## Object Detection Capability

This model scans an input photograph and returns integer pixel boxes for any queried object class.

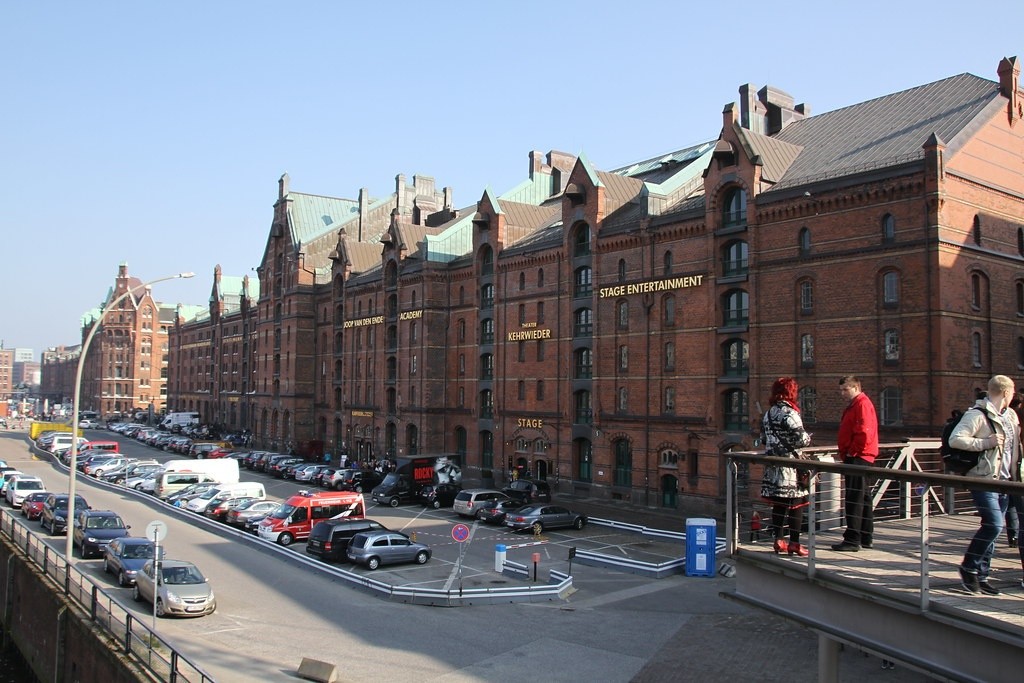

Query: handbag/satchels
[796,456,819,490]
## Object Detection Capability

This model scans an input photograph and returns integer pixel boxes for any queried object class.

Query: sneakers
[979,582,999,595]
[958,567,980,592]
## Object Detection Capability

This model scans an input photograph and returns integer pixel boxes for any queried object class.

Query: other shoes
[1008,537,1018,548]
[832,539,861,551]
[862,542,873,547]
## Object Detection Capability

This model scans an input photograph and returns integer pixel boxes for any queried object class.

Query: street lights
[65,272,196,562]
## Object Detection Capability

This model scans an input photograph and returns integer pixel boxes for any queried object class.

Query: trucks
[158,412,199,429]
[372,452,462,507]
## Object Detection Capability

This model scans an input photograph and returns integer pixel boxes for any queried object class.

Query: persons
[1000,392,1024,587]
[508,468,531,484]
[179,421,257,450]
[947,375,1022,596]
[831,378,878,551]
[449,458,462,487]
[761,378,811,556]
[33,407,56,422]
[750,511,760,541]
[286,444,383,482]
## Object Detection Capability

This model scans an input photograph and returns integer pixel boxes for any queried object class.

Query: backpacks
[940,408,997,475]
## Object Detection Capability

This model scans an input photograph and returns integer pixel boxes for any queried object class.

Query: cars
[102,537,167,587]
[452,489,513,520]
[417,484,465,509]
[476,498,524,524]
[79,409,386,493]
[165,481,282,534]
[133,559,216,619]
[346,532,432,570]
[306,518,411,564]
[504,503,589,536]
[35,430,162,485]
[501,479,551,504]
[0,459,131,559]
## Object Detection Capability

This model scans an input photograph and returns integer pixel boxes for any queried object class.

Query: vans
[257,491,365,547]
[153,472,216,501]
[140,458,239,493]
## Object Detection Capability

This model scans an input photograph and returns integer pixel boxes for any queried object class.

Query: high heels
[774,539,788,554]
[787,541,808,556]
